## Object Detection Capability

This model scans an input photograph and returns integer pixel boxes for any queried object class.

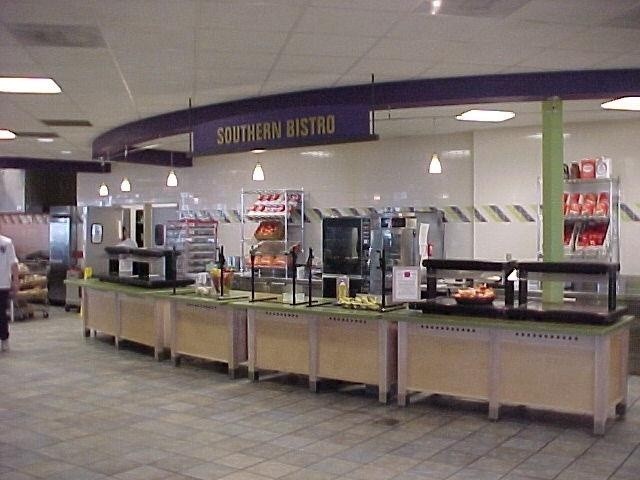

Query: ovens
[319,215,382,297]
[48,200,122,308]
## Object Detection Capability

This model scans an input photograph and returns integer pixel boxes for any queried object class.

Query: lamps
[252,153,265,181]
[99,156,109,196]
[166,151,178,186]
[120,144,130,192]
[429,117,442,174]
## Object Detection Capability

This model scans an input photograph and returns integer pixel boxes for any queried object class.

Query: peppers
[339,295,380,311]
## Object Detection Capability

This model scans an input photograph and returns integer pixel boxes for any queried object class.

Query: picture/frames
[90,223,103,244]
[392,265,422,303]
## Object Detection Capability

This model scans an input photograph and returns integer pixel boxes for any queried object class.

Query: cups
[195,273,234,296]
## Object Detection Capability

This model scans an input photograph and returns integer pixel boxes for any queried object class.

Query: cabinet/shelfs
[160,219,218,277]
[537,177,622,294]
[240,188,307,278]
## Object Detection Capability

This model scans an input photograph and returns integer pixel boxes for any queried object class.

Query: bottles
[339,280,346,300]
[563,160,580,180]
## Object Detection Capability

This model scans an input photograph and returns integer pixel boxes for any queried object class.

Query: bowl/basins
[452,296,495,305]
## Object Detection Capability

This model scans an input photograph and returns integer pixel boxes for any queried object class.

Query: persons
[1,235,19,351]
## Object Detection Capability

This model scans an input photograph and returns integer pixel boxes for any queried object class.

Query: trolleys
[7,259,51,321]
[64,270,100,312]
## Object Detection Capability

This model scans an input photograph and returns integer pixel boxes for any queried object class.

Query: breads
[458,283,494,299]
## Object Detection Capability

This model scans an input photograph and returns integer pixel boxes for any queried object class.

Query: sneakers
[1,339,9,352]
